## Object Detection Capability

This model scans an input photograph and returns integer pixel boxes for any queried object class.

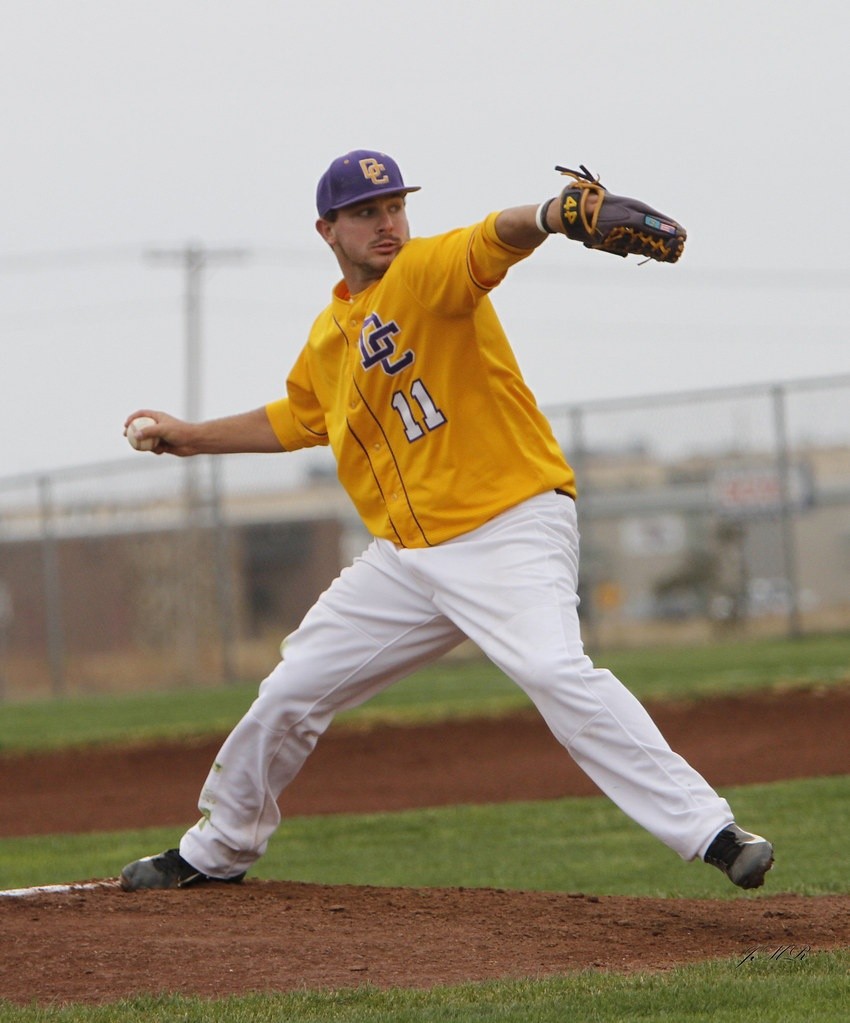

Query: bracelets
[536,196,557,234]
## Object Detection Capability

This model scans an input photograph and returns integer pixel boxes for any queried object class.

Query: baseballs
[127,418,160,450]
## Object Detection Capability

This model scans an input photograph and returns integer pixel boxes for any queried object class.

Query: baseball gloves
[555,164,688,271]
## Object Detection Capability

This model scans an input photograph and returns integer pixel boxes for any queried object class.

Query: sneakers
[122,848,247,893]
[704,823,774,890]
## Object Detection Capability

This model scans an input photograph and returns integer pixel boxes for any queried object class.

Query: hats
[316,150,421,219]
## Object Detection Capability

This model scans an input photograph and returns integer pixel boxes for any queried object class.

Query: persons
[121,150,777,894]
